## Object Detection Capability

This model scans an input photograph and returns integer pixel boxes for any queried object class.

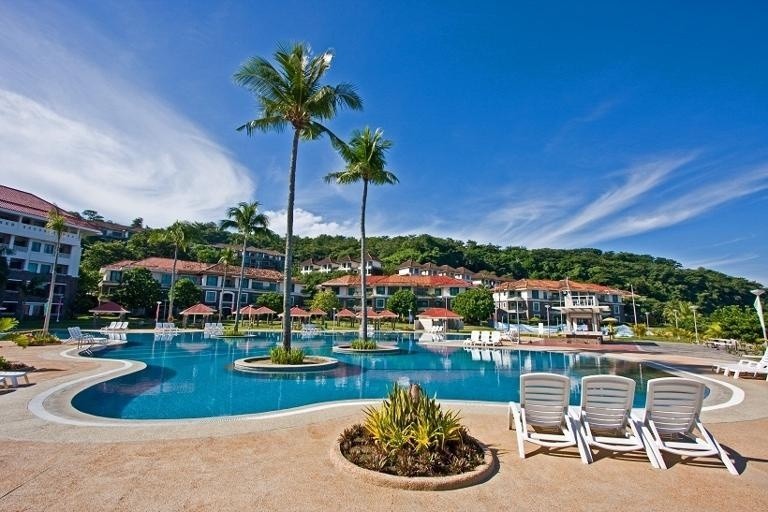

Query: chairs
[572,374,659,471]
[634,375,738,478]
[506,371,588,463]
[63,326,95,349]
[155,323,177,331]
[101,321,130,331]
[0,371,29,392]
[710,345,768,381]
[463,329,503,345]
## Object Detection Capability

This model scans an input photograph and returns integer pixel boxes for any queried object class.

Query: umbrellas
[602,316,618,342]
